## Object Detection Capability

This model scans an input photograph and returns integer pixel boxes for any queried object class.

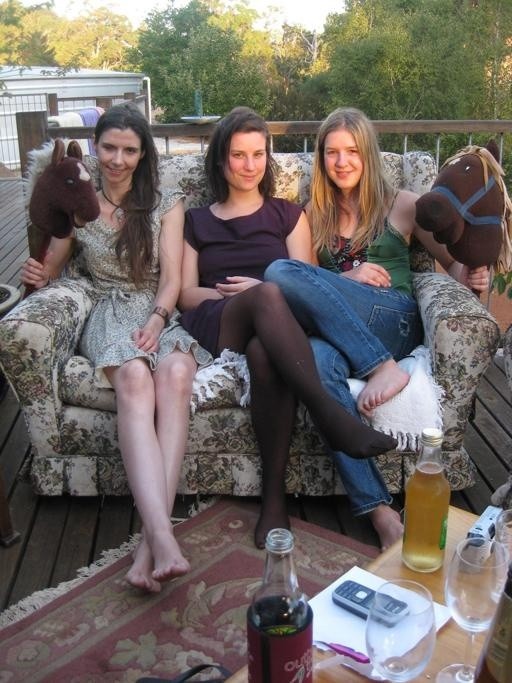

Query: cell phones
[332,580,409,626]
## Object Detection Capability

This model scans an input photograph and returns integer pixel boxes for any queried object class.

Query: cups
[491,508,512,604]
[365,580,436,683]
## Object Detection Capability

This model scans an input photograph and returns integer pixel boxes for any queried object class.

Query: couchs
[1,149,501,509]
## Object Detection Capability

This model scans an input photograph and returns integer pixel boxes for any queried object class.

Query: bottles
[246,528,313,683]
[473,562,512,683]
[401,428,450,573]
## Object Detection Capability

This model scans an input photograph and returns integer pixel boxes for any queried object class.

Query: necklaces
[101,187,130,223]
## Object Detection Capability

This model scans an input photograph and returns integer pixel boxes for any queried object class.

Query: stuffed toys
[26,135,100,293]
[414,145,511,278]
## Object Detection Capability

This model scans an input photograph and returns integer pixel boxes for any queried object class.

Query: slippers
[136,664,232,682]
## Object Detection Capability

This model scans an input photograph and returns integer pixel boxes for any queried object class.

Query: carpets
[0,493,388,683]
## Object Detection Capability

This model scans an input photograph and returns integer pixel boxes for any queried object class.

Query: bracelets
[152,306,171,328]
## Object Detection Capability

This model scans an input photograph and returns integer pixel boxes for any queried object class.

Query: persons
[262,106,492,552]
[18,99,213,593]
[174,105,400,549]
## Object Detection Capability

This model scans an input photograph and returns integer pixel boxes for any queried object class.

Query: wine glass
[435,537,510,683]
